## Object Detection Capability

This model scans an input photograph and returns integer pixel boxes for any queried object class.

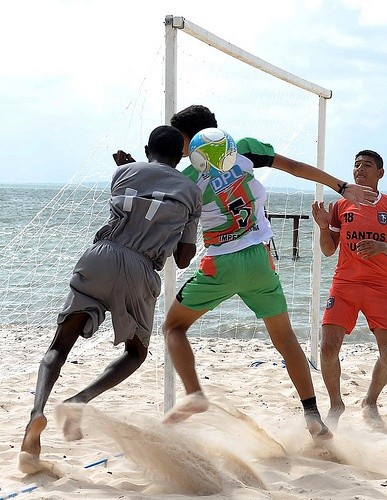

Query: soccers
[188,128,238,177]
